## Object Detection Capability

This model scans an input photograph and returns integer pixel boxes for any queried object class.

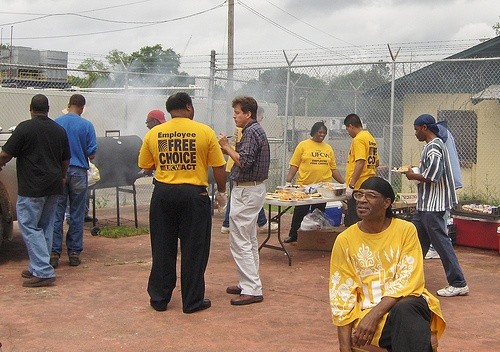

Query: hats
[148,109,166,124]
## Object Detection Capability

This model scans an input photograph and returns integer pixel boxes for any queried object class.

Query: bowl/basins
[286,187,306,192]
[317,183,346,197]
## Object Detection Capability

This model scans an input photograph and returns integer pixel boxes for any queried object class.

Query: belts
[233,180,264,186]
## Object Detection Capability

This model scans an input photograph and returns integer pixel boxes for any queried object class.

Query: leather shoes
[231,293,264,305]
[284,236,296,243]
[226,285,241,294]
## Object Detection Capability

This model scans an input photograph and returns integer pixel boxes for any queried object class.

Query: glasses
[352,190,376,200]
[145,121,153,124]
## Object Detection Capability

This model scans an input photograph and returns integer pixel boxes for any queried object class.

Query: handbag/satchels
[87,157,101,187]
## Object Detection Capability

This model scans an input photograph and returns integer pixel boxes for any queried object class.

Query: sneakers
[436,285,469,297]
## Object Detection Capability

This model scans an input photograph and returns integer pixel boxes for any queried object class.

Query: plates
[390,170,408,172]
[266,193,322,202]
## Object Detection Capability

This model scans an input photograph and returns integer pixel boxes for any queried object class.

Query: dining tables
[258,192,356,266]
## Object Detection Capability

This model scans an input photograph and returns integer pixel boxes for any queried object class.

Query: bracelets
[349,184,354,189]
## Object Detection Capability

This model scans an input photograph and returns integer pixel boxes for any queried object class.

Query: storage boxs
[297,226,348,251]
[452,215,500,251]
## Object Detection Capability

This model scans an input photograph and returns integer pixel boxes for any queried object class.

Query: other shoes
[50,251,59,267]
[69,251,81,266]
[85,215,98,222]
[423,250,440,259]
[258,222,278,234]
[23,275,56,287]
[197,299,211,311]
[221,226,230,234]
[22,269,35,278]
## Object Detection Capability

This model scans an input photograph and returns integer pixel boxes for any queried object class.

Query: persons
[283,122,345,242]
[425,121,463,258]
[330,177,445,352]
[137,110,167,171]
[222,107,280,234]
[405,114,470,297]
[343,114,380,225]
[47,94,97,268]
[218,96,271,306]
[138,92,227,315]
[0,94,71,287]
